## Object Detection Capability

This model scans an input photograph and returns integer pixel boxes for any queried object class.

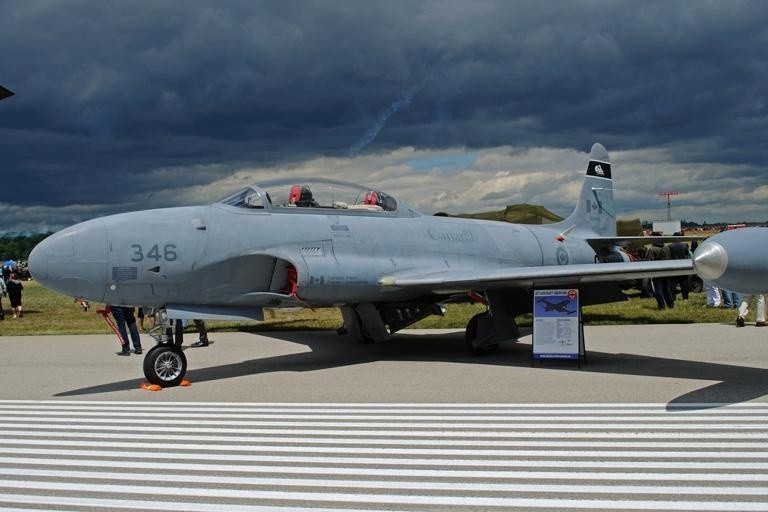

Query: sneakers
[117,351,131,355]
[755,321,768,326]
[737,316,745,326]
[135,347,143,354]
[192,340,208,347]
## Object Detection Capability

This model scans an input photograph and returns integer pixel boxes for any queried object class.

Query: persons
[138,304,145,331]
[148,307,156,329]
[0,277,8,321]
[0,259,31,284]
[643,228,768,327]
[6,273,24,320]
[174,319,209,347]
[110,304,142,356]
